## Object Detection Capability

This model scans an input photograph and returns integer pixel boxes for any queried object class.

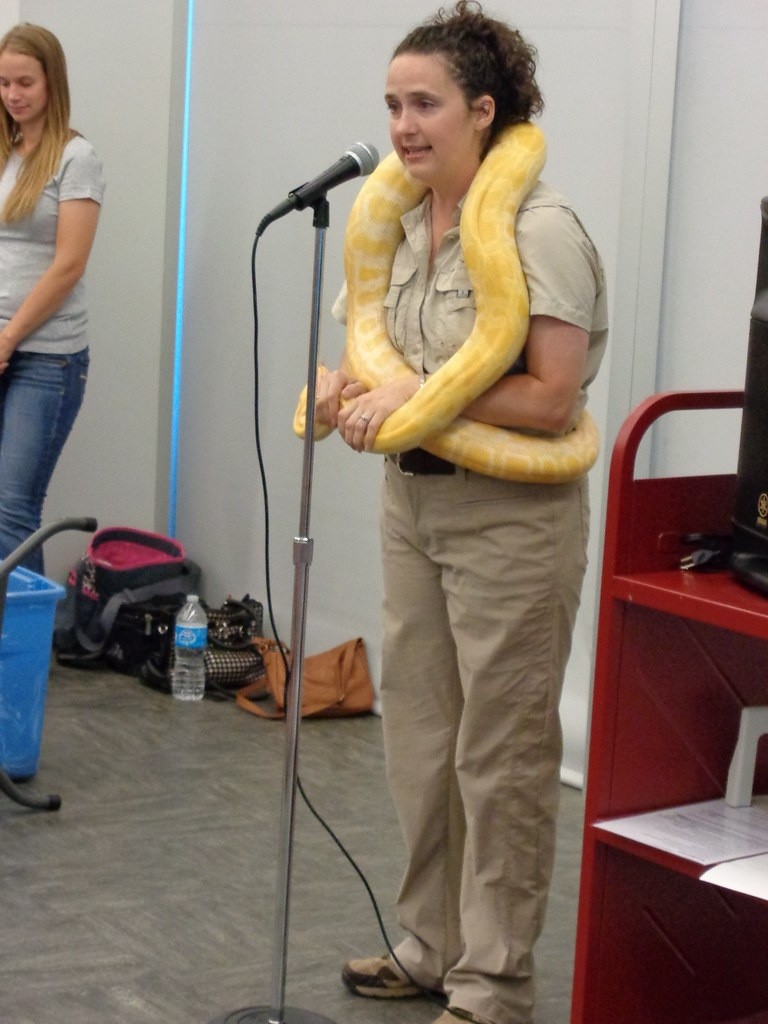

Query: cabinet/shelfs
[571,388,768,1024]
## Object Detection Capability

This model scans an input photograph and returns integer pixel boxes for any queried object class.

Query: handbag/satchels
[140,594,267,695]
[52,528,204,676]
[237,637,377,720]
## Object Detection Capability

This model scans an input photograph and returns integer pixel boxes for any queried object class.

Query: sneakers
[342,950,428,998]
[433,1005,492,1024]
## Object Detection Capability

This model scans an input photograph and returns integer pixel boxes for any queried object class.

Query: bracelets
[419,375,426,388]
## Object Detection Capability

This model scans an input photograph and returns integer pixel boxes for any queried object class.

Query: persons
[0,23,106,576]
[315,0,610,1024]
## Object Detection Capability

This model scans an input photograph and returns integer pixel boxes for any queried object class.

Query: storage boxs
[0,564,68,779]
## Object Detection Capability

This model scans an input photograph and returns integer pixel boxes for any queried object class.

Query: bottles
[171,595,209,702]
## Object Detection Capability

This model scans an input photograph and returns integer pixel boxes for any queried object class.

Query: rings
[317,395,319,399]
[360,415,370,423]
